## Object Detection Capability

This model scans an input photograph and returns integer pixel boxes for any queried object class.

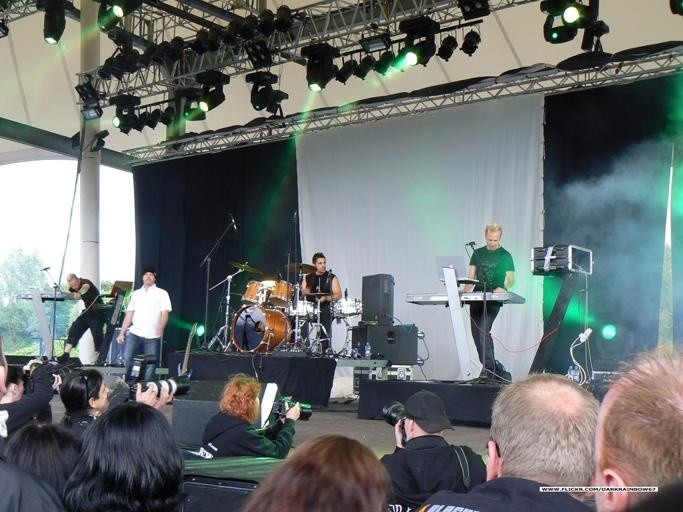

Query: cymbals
[228,262,263,278]
[284,262,318,275]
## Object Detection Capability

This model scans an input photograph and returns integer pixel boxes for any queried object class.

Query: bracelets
[502,288,507,291]
[77,291,82,296]
[157,321,165,329]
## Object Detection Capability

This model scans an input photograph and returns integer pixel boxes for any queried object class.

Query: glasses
[74,367,91,400]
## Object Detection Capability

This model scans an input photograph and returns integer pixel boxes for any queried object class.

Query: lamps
[0,0,682,154]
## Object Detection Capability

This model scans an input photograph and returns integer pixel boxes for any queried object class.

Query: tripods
[40,273,76,367]
[304,295,338,359]
[175,223,309,352]
[334,315,364,359]
[457,245,509,386]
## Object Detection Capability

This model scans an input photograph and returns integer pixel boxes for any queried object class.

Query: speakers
[173,400,261,451]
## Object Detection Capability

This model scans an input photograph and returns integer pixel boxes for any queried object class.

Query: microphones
[465,240,477,245]
[118,337,125,343]
[325,269,332,287]
[40,266,50,272]
[229,213,237,230]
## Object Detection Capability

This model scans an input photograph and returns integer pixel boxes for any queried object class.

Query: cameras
[383,401,407,439]
[272,397,312,421]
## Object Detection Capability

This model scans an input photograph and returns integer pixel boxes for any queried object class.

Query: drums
[240,280,268,305]
[268,278,295,308]
[230,303,292,354]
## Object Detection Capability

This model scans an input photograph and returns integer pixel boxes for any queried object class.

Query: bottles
[573,366,579,383]
[567,366,573,381]
[363,341,371,360]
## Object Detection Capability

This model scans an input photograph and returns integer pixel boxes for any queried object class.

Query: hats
[404,390,457,435]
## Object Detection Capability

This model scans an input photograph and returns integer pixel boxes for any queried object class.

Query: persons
[300,252,341,355]
[458,222,515,382]
[113,268,171,385]
[58,273,105,357]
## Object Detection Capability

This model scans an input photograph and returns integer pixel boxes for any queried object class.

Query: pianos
[404,291,526,305]
[16,293,69,302]
